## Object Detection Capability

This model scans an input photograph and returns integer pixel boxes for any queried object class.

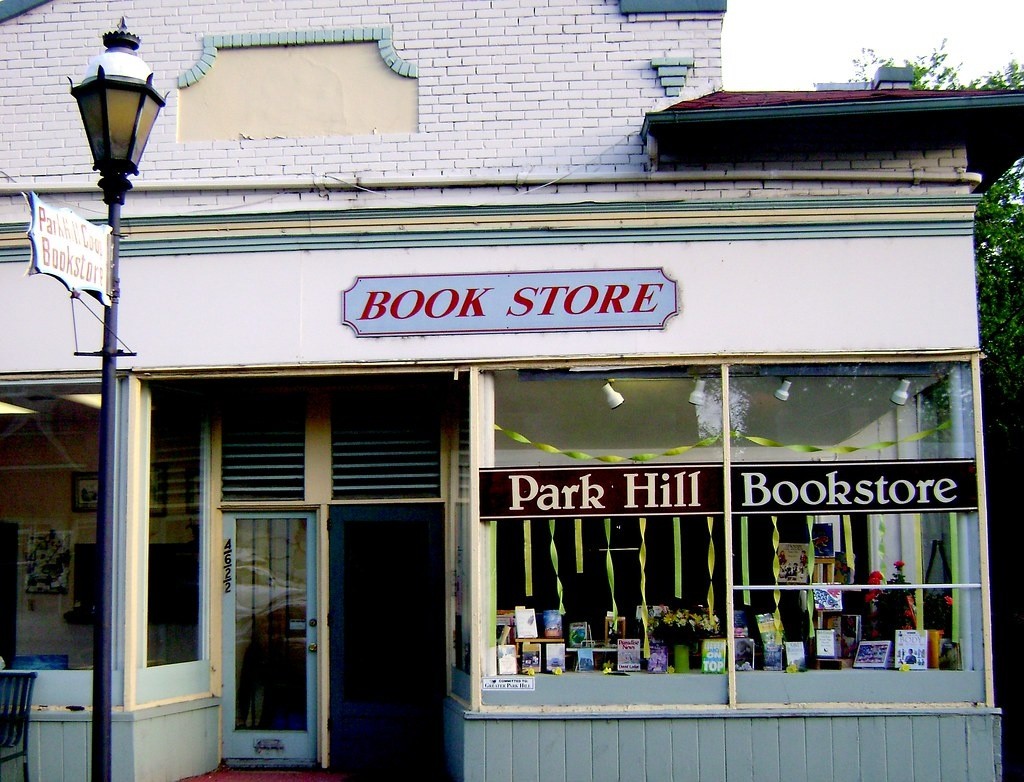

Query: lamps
[600,379,625,411]
[773,378,793,402]
[889,379,911,406]
[688,377,708,406]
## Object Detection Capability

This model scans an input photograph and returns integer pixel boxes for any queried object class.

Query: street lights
[63,16,172,782]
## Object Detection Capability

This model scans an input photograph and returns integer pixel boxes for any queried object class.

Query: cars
[235,553,307,711]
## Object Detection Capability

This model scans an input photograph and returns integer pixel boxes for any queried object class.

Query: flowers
[646,602,721,638]
[865,559,952,629]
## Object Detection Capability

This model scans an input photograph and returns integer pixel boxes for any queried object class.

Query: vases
[674,643,692,673]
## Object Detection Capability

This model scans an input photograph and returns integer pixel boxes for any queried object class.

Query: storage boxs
[805,523,836,558]
[852,639,893,669]
[701,638,727,675]
[646,645,669,674]
[735,638,756,672]
[617,638,641,673]
[814,628,838,659]
[777,543,811,585]
[928,630,960,671]
[811,582,843,610]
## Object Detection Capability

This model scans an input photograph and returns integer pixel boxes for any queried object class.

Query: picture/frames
[70,469,100,515]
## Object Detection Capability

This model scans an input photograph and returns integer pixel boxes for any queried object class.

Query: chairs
[0,671,38,782]
